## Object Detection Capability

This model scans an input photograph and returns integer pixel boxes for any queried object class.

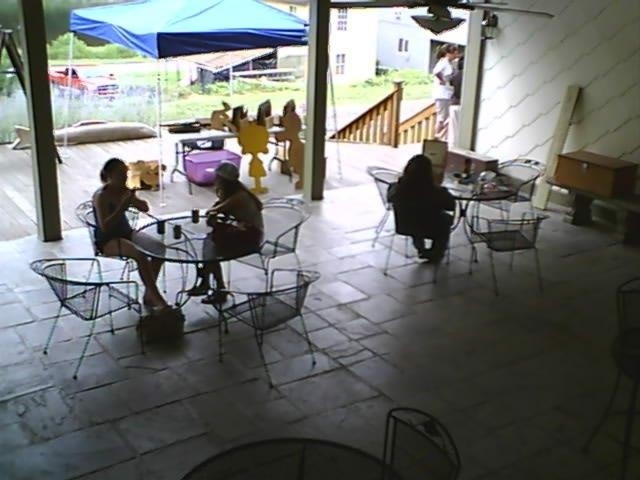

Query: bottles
[451,157,490,196]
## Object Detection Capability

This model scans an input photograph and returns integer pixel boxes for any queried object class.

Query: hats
[205,160,240,183]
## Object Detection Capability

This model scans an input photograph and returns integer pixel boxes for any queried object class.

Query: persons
[384,154,456,262]
[92,158,168,307]
[432,43,460,138]
[186,162,264,304]
[435,57,464,138]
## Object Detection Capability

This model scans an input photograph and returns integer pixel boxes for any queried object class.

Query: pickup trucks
[50,63,122,103]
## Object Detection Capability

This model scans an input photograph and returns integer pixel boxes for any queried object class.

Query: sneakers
[187,285,209,295]
[145,296,181,318]
[416,248,443,264]
[201,293,227,304]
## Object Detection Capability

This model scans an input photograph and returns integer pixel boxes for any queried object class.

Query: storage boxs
[554,149,640,198]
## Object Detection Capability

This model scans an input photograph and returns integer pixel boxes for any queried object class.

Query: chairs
[217,269,321,391]
[382,406,461,478]
[75,200,166,311]
[29,258,145,380]
[212,194,312,293]
[364,158,551,298]
[582,275,640,480]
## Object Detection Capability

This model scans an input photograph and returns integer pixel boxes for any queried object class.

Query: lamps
[480,13,498,40]
[412,4,465,37]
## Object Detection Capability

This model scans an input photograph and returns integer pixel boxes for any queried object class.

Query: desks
[166,124,305,196]
[131,216,259,335]
[177,436,400,479]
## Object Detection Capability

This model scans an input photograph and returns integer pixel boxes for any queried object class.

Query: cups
[157,219,165,235]
[172,224,182,239]
[192,208,200,224]
[209,212,218,225]
